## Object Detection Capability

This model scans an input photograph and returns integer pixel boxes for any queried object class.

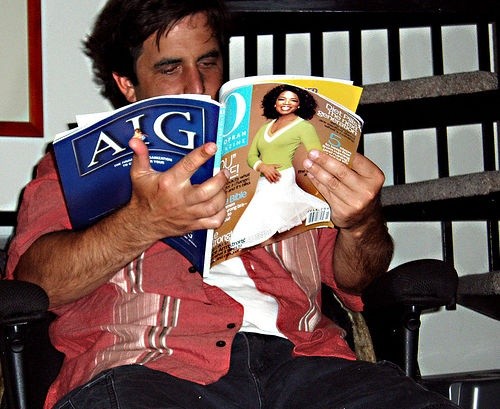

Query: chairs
[0,257,459,409]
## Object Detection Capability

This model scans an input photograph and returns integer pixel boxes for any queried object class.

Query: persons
[5,0,459,409]
[229,83,330,248]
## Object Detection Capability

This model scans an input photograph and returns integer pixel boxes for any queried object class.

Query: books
[52,75,364,278]
